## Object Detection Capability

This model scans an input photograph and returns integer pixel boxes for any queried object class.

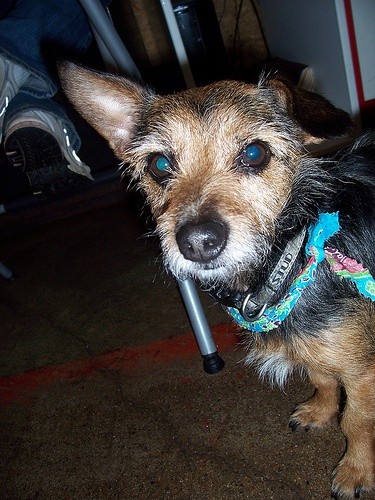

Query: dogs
[57,56,375,500]
